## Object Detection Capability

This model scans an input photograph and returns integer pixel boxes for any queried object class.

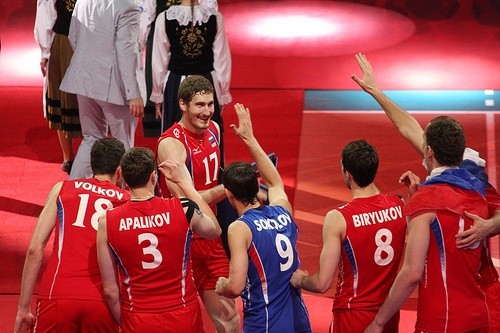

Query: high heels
[62,161,72,175]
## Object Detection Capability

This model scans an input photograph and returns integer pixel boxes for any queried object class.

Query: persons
[155,74,241,333]
[215,102,312,333]
[289,141,421,333]
[34,0,233,181]
[97,147,222,332]
[14,136,131,332]
[350,51,500,333]
